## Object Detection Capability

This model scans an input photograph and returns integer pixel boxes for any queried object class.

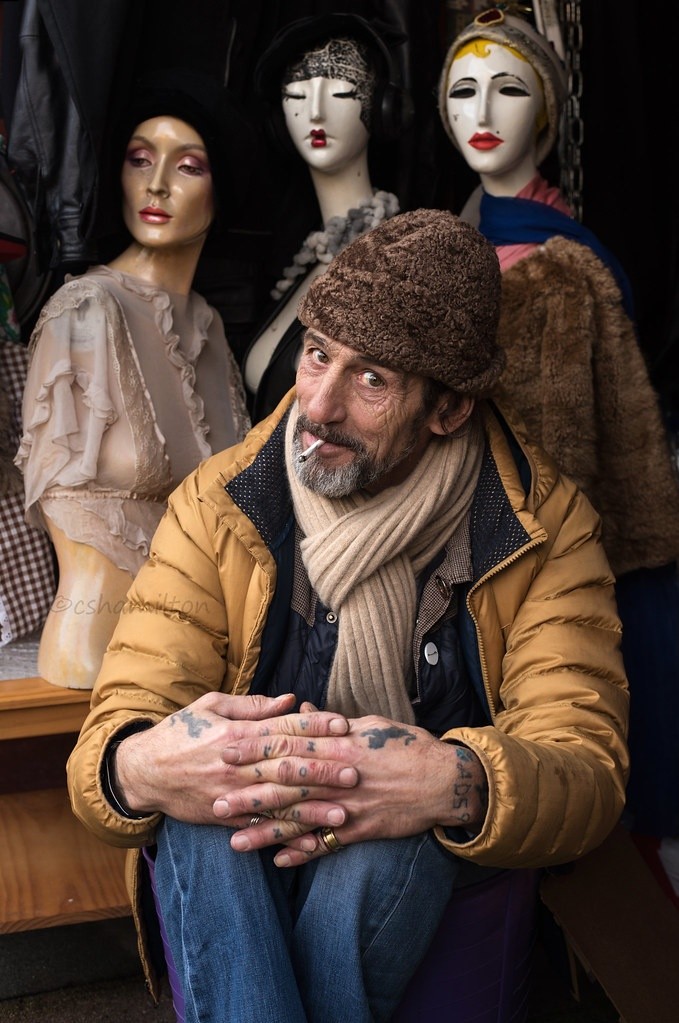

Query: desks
[0,626,92,740]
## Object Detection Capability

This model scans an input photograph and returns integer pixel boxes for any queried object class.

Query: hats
[297,208,505,395]
[437,9,565,169]
[112,66,260,253]
[254,9,427,194]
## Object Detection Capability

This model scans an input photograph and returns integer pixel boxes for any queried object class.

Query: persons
[439,8,679,576]
[66,211,628,1023]
[240,15,401,427]
[12,95,253,691]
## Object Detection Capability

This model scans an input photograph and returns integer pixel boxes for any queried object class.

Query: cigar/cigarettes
[297,438,325,462]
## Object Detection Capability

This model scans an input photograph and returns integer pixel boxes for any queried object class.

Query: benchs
[0,732,138,936]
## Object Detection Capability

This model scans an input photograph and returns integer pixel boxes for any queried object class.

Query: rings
[249,814,261,827]
[321,828,344,851]
